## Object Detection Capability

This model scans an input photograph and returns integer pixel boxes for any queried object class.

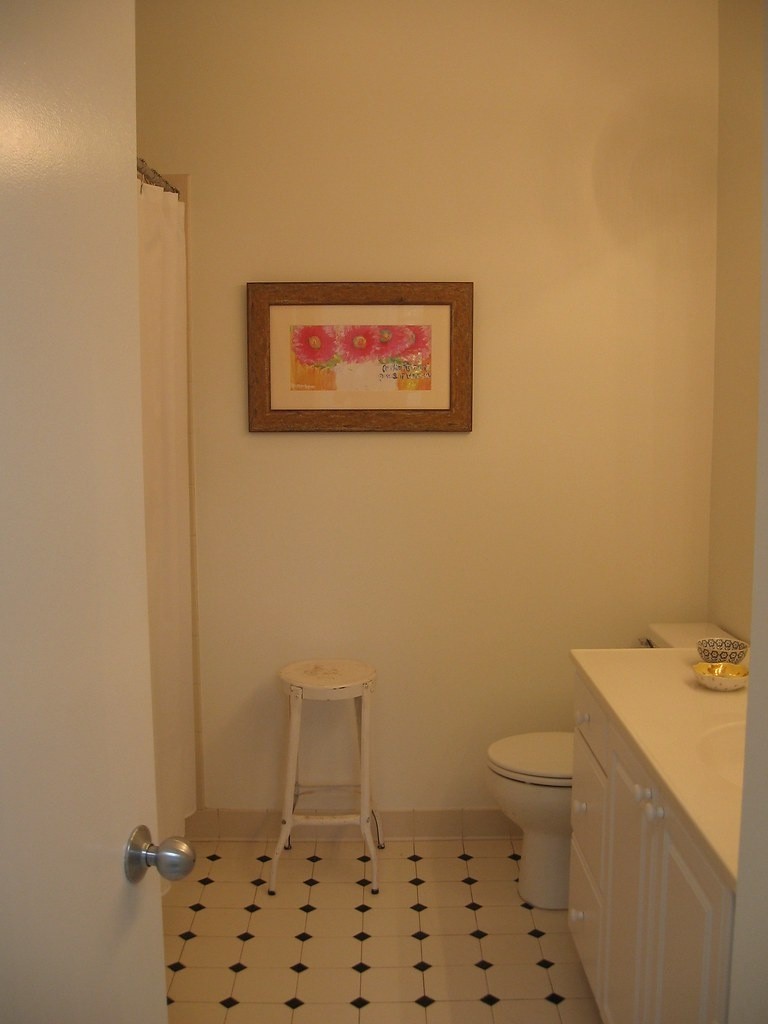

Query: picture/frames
[247,282,474,433]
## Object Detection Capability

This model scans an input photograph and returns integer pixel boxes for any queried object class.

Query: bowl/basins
[696,638,750,665]
[692,662,750,692]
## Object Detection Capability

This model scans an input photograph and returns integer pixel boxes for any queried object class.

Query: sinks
[689,718,743,785]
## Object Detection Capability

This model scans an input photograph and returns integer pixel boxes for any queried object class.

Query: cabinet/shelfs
[569,646,750,1024]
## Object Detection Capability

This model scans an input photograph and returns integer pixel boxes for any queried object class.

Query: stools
[267,660,384,899]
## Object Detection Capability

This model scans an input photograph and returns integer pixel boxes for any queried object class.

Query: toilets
[486,623,739,909]
